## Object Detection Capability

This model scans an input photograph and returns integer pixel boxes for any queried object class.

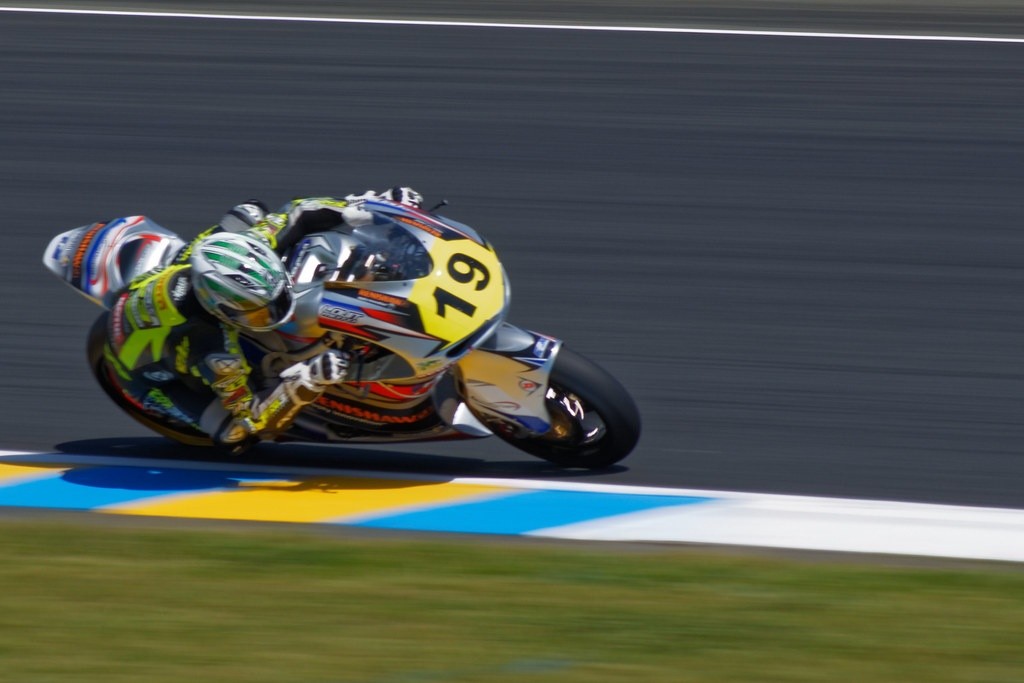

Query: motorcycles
[43,196,644,470]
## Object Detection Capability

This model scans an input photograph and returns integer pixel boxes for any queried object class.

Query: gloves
[280,348,351,405]
[340,187,424,227]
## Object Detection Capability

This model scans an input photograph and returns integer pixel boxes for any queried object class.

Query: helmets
[190,232,297,333]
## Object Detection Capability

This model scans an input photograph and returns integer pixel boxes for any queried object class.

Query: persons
[101,185,426,460]
